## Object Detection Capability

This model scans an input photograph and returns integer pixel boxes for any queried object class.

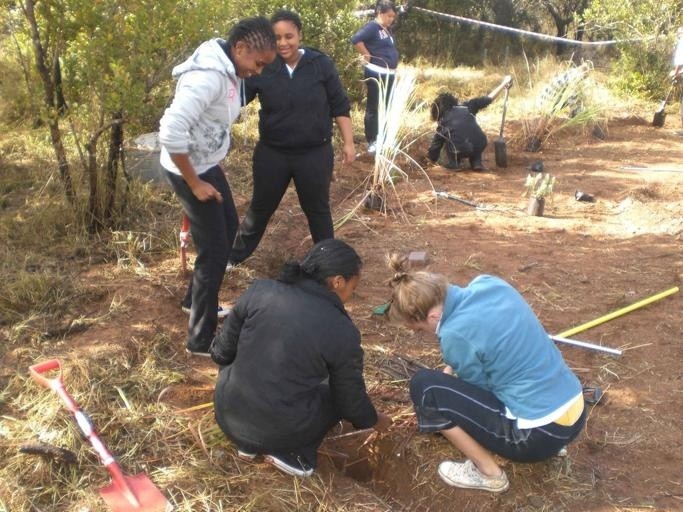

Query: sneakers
[266,452,315,476]
[437,459,509,493]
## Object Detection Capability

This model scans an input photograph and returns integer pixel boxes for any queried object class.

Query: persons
[158,15,278,358]
[670,27,683,84]
[211,239,393,478]
[350,2,400,149]
[227,10,355,268]
[426,75,512,172]
[383,249,586,494]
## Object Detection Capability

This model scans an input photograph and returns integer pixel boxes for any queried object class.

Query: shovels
[653,65,683,127]
[494,82,513,168]
[27,357,176,511]
